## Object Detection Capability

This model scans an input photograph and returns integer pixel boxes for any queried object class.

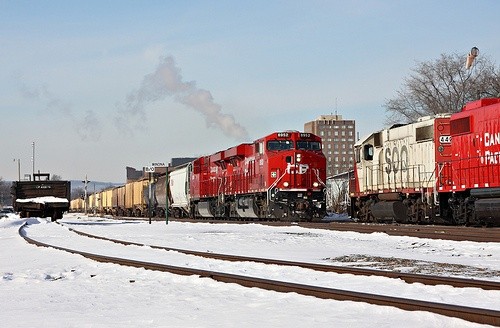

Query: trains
[69,129,328,224]
[347,96,500,229]
